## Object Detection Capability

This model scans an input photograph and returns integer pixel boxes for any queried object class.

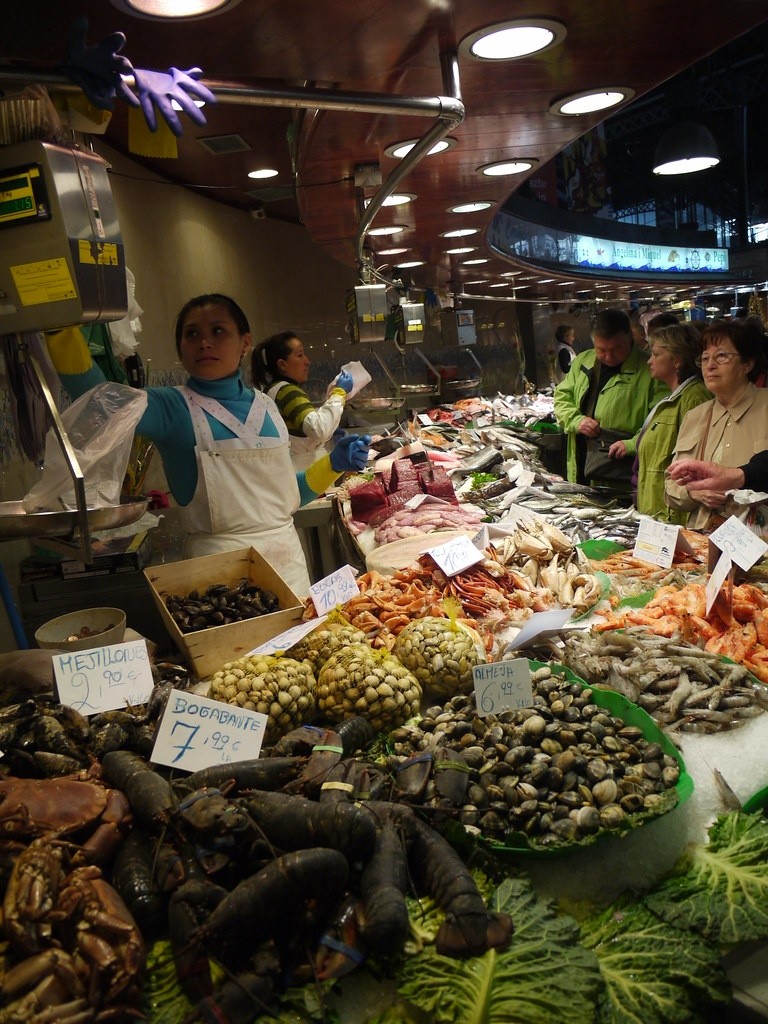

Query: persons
[549,322,580,392]
[663,446,768,496]
[647,314,680,353]
[37,291,375,614]
[664,320,768,537]
[629,320,650,360]
[635,323,720,530]
[250,329,356,501]
[553,307,670,501]
[685,306,768,373]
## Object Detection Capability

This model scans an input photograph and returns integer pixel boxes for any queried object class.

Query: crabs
[0,748,146,1024]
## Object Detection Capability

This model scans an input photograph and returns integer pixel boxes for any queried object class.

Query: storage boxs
[143,545,307,681]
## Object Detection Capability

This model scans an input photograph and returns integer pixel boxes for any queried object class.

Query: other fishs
[437,425,641,549]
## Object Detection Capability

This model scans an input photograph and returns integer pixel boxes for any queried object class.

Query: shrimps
[713,768,742,810]
[534,528,767,734]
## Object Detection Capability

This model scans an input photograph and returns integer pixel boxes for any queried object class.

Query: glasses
[695,349,741,368]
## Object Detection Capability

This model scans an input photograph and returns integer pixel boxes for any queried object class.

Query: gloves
[331,433,372,470]
[336,369,353,394]
[333,428,347,444]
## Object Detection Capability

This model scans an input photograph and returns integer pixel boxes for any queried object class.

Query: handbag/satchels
[583,426,637,481]
